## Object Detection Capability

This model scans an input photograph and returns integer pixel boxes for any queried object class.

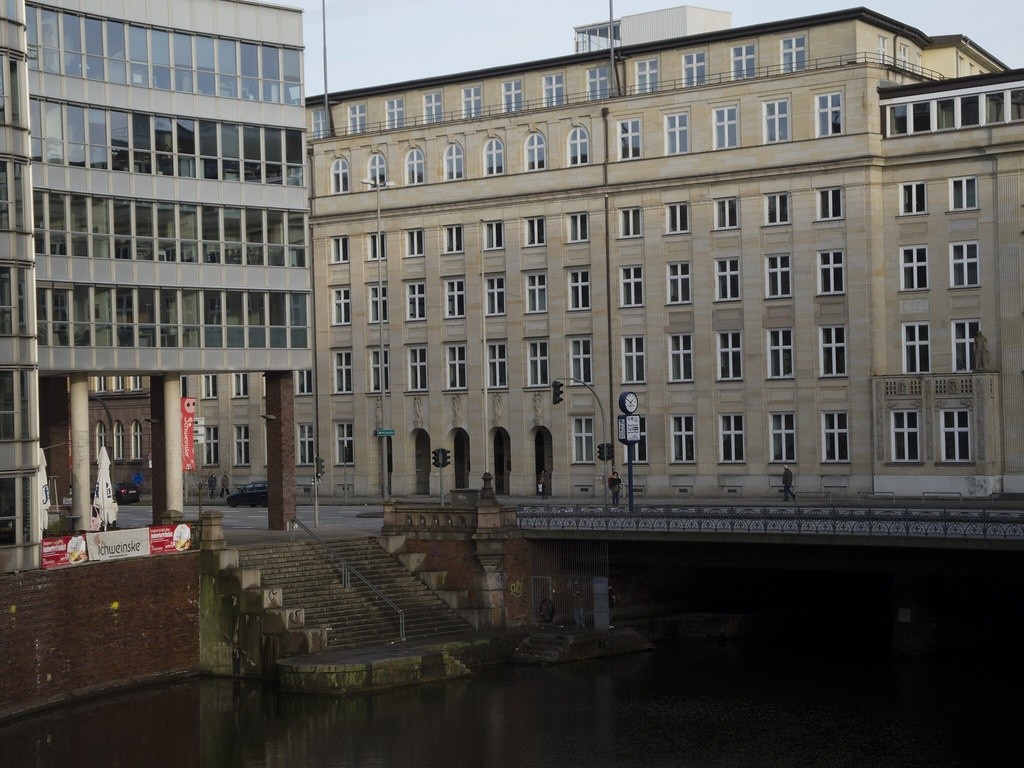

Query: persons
[4,505,16,528]
[220,471,230,498]
[208,473,217,499]
[538,470,548,499]
[133,469,144,494]
[608,472,621,508]
[782,464,796,502]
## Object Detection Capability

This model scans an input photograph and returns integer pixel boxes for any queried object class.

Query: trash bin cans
[449,489,481,511]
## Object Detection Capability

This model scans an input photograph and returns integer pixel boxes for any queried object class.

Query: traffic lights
[605,442,613,461]
[441,447,451,468]
[315,456,326,478]
[551,380,564,406]
[432,449,440,469]
[597,444,605,461]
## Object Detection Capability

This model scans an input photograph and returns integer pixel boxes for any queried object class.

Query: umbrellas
[93,445,119,532]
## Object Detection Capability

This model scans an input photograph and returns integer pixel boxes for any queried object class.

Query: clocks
[619,392,639,414]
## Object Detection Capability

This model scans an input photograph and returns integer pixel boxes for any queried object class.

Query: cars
[113,482,139,506]
[226,481,269,509]
